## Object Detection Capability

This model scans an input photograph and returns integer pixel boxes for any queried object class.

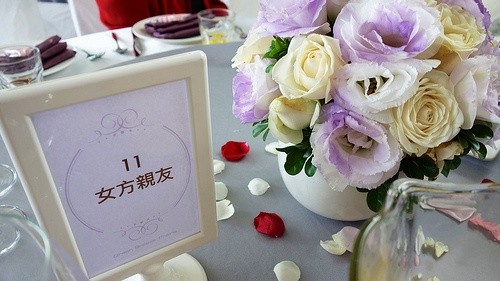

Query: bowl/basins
[132,14,201,45]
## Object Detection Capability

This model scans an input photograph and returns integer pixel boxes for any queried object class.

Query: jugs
[350,176,498,281]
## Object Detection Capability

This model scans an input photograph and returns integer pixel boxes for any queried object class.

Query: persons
[94,0,229,31]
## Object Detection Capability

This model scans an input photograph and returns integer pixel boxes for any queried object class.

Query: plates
[134,37,155,56]
[42,55,77,77]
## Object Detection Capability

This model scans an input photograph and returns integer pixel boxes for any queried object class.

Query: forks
[75,45,105,62]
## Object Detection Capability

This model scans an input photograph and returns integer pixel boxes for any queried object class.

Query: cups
[0,45,45,91]
[198,8,237,45]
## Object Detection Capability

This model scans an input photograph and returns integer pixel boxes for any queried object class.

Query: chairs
[68,0,230,38]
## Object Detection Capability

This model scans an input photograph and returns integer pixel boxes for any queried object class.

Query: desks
[0,0,500,281]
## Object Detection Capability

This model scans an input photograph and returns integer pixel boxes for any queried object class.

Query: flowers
[230,0,500,193]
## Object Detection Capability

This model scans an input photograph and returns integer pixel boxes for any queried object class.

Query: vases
[272,121,434,224]
